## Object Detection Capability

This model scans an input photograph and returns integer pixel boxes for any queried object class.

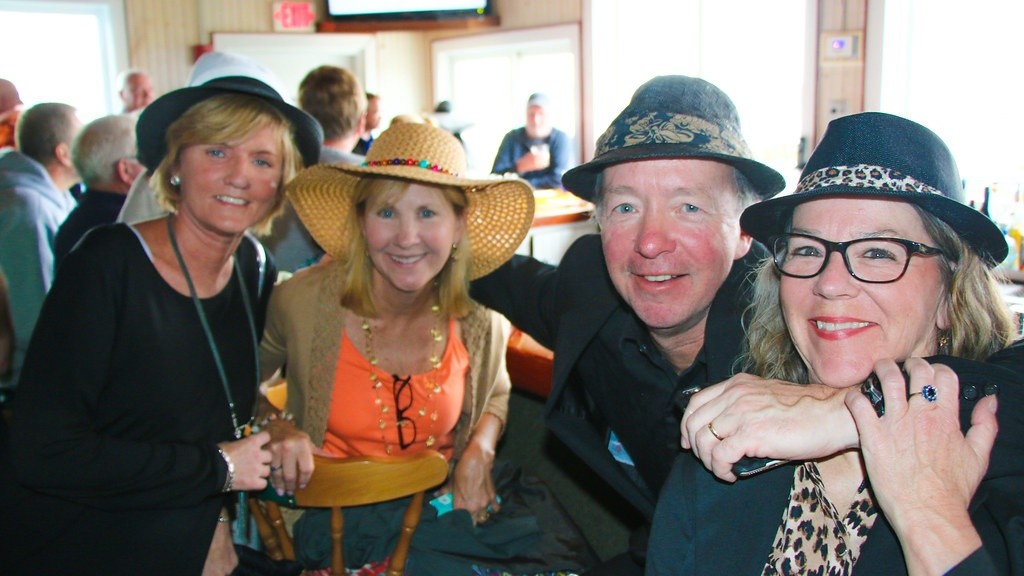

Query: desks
[529,189,599,259]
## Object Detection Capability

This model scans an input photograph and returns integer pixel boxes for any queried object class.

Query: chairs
[248,452,449,576]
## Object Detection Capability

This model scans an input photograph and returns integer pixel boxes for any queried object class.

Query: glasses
[391,374,417,449]
[768,233,944,284]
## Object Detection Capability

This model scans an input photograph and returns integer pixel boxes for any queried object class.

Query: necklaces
[360,279,443,455]
[167,211,260,439]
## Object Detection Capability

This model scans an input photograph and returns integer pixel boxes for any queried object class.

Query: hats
[134,77,326,177]
[528,93,548,107]
[188,51,279,93]
[284,123,536,283]
[560,75,786,203]
[737,111,1010,272]
[0,79,24,115]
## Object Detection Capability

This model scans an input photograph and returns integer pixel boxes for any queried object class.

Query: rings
[477,511,490,523]
[909,385,938,402]
[707,420,724,442]
[269,464,283,472]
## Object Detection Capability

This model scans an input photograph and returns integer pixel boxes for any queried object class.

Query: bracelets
[860,377,885,418]
[251,412,294,434]
[218,446,234,492]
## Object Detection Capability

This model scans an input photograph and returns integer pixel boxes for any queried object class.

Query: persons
[351,92,383,156]
[467,75,1024,576]
[252,65,368,272]
[490,92,570,190]
[251,122,536,576]
[0,51,267,401]
[0,74,325,576]
[645,112,1023,576]
